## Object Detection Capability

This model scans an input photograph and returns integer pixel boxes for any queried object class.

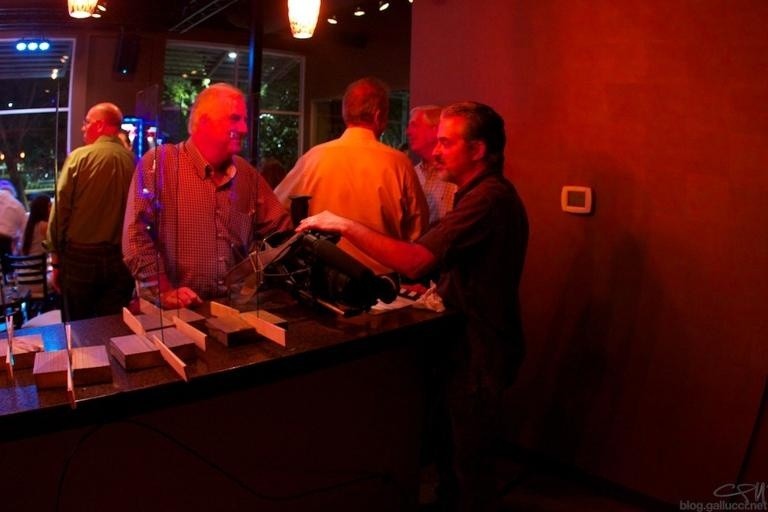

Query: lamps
[67,0,98,19]
[327,0,413,26]
[287,0,321,40]
[16,31,50,52]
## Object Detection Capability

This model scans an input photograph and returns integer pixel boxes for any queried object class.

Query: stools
[0,285,31,330]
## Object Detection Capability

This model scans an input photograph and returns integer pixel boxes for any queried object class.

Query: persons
[2,103,141,308]
[270,78,457,279]
[121,83,294,308]
[294,102,529,512]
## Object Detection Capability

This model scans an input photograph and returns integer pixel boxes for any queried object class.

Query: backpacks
[222,227,400,318]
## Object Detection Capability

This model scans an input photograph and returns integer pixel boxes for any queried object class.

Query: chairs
[0,252,67,322]
